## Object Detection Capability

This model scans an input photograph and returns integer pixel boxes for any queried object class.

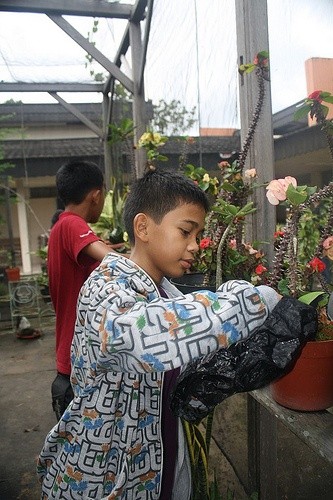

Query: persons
[36,169,283,500]
[51,209,126,249]
[47,162,131,424]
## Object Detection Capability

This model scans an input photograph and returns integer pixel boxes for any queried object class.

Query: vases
[271,329,333,412]
[168,273,216,294]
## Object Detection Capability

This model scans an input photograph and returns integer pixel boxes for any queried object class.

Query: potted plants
[0,250,20,281]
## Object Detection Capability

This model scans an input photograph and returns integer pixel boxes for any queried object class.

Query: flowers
[135,125,174,171]
[183,50,271,282]
[250,89,332,333]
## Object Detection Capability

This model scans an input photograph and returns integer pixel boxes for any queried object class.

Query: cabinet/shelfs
[6,277,47,339]
